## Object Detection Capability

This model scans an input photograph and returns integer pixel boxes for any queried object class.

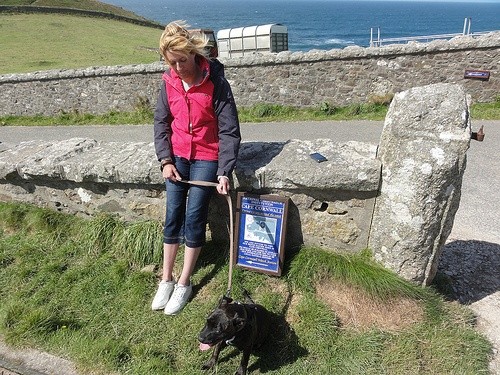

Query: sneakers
[151,275,176,310]
[163,280,193,315]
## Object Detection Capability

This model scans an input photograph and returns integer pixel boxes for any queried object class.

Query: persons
[151,21,240,315]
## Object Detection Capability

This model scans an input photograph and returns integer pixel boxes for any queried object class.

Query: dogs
[197,291,273,375]
